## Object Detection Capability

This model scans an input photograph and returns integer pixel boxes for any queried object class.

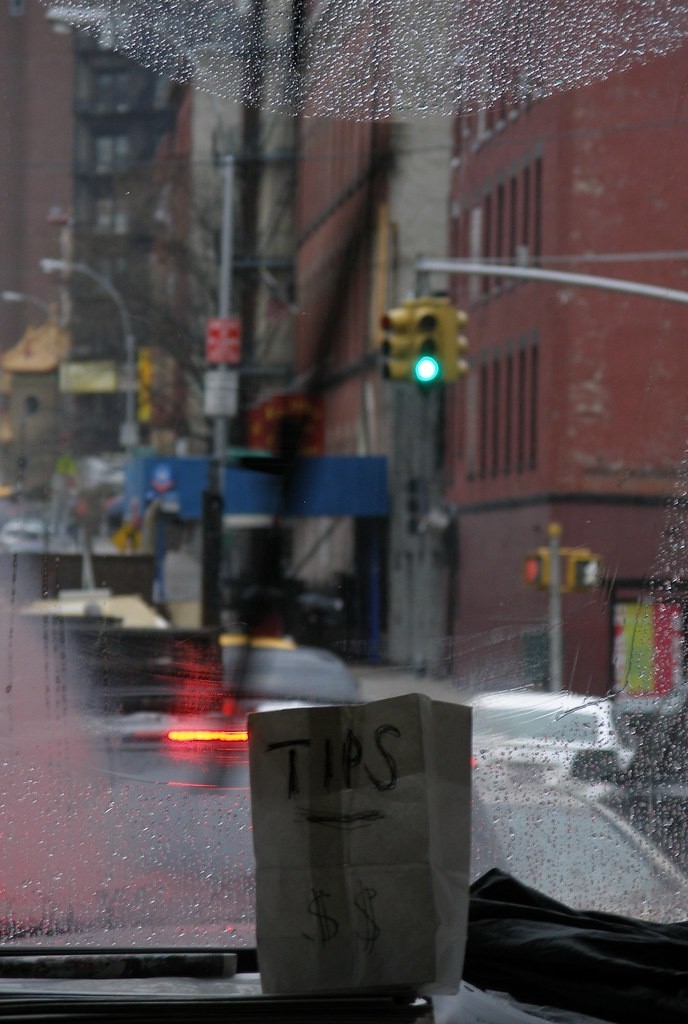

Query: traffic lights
[443,305,470,386]
[381,308,409,383]
[569,552,600,592]
[524,547,551,591]
[408,306,447,383]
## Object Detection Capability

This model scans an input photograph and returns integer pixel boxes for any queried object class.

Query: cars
[105,628,366,834]
[465,685,636,815]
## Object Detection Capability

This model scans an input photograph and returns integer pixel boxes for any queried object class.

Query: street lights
[37,254,140,458]
[3,291,65,471]
